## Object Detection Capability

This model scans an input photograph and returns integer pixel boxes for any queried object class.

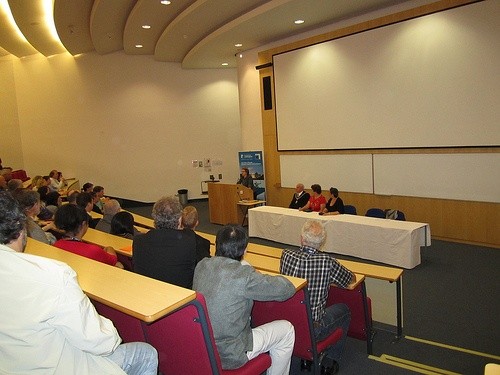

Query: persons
[278,220,356,375]
[0,164,158,375]
[289,183,310,210]
[237,168,257,200]
[132,195,195,290]
[50,204,118,267]
[299,184,327,212]
[191,225,297,375]
[181,206,211,264]
[321,187,345,214]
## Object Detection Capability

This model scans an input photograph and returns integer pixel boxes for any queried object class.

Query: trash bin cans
[178,189,187,205]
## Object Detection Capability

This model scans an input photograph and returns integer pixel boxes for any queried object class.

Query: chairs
[89,299,145,345]
[251,288,342,375]
[384,209,405,220]
[341,205,357,215]
[365,208,384,219]
[11,170,30,182]
[141,292,272,375]
[326,282,378,354]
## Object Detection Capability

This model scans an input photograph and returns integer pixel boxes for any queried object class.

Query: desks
[22,178,404,375]
[249,206,432,269]
[237,199,267,226]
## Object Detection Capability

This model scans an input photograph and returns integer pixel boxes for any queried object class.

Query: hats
[103,199,120,215]
[46,191,61,200]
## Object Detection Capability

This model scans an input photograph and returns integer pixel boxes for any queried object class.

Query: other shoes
[319,358,335,375]
[301,359,314,373]
